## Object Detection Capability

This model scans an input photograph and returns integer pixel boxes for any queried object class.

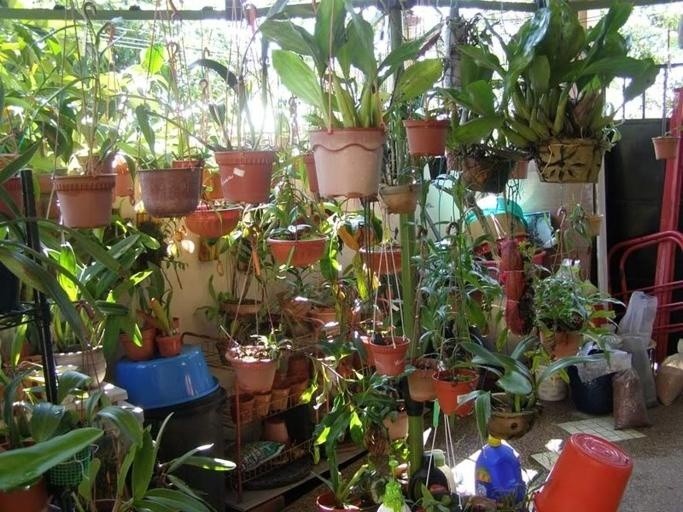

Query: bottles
[410,447,457,505]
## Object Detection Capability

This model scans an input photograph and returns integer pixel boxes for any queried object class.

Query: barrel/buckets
[407,453,451,507]
[428,449,458,495]
[475,432,526,507]
[534,359,568,402]
[526,432,633,512]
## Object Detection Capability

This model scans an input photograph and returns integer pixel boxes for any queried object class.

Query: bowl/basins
[465,196,528,231]
[114,342,220,408]
